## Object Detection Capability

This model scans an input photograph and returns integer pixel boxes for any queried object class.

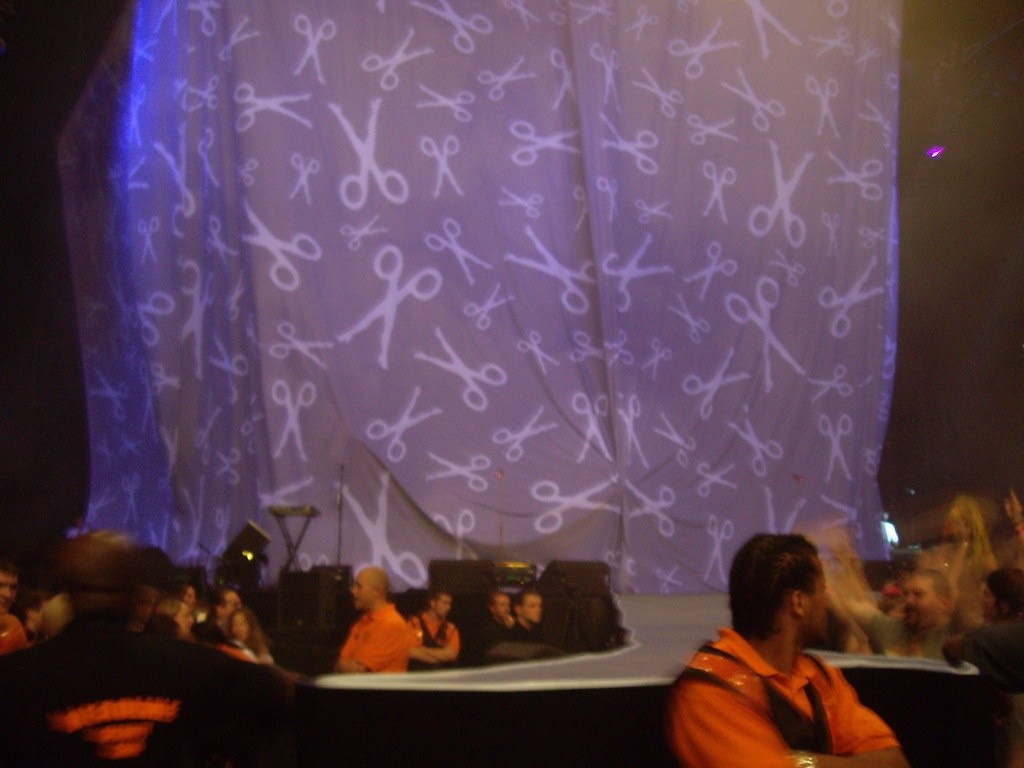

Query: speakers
[536,559,611,598]
[278,571,349,633]
[430,559,499,599]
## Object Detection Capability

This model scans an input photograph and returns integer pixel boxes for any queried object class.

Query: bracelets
[792,750,818,768]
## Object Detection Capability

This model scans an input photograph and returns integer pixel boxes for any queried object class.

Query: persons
[660,532,910,768]
[406,588,461,671]
[790,482,1024,700]
[0,529,293,767]
[464,587,545,664]
[335,565,409,672]
[1,558,274,665]
